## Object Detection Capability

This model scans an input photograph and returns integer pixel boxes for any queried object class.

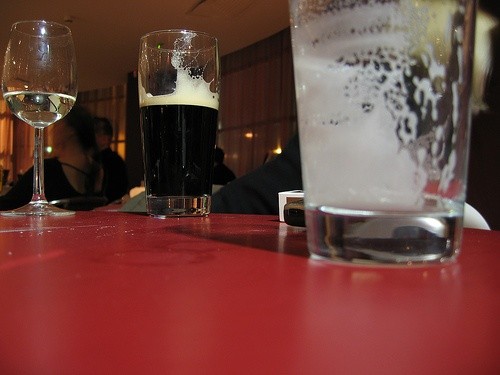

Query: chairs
[49,197,109,211]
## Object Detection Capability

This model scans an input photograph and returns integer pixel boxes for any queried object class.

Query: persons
[213,132,303,216]
[91,116,129,201]
[136,147,236,189]
[0,104,113,214]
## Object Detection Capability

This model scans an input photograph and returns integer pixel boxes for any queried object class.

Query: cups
[289,0,475,267]
[137,29,221,217]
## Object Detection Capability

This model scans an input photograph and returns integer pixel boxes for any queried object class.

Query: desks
[0,207,500,375]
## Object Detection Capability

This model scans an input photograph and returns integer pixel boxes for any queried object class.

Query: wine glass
[1,20,77,217]
